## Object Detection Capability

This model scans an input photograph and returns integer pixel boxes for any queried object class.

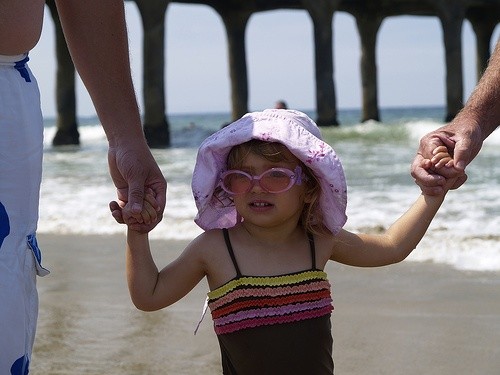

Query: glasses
[218,163,309,194]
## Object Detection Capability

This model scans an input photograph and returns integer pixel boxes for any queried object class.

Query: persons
[0,0,167,375]
[121,108,459,375]
[411,37,500,195]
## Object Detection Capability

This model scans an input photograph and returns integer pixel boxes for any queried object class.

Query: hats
[192,108,348,233]
